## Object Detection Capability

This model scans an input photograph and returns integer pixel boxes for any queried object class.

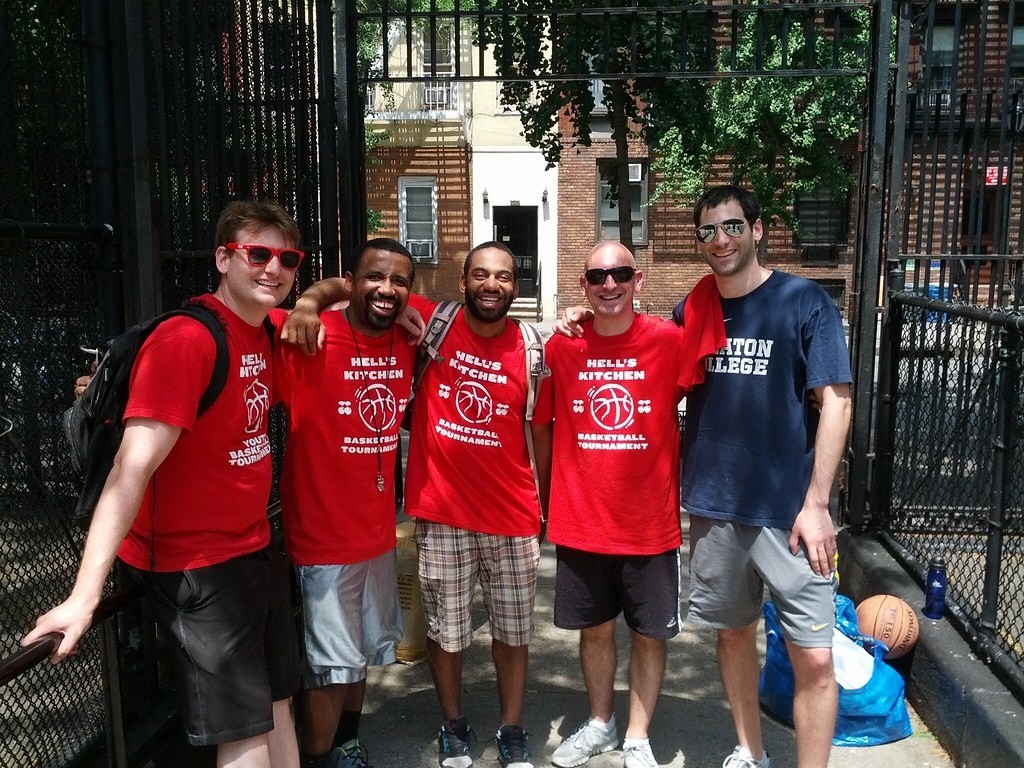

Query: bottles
[924,556,947,619]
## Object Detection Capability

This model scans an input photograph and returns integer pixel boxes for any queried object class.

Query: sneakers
[548,715,619,768]
[496,722,536,768]
[438,719,474,767]
[624,737,660,767]
[328,739,373,767]
[725,746,770,768]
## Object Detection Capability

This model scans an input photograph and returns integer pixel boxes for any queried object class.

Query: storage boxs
[396,515,429,665]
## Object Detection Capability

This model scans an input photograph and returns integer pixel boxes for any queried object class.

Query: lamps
[542,188,548,203]
[482,188,488,203]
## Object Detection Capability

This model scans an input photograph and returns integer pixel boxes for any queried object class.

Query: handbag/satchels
[762,593,914,747]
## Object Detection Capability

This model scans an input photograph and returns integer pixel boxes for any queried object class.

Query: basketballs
[855,594,919,659]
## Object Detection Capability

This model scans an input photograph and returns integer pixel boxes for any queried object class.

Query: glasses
[586,267,636,284]
[696,218,752,238]
[226,243,304,271]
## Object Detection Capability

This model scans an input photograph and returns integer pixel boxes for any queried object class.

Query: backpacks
[67,309,158,522]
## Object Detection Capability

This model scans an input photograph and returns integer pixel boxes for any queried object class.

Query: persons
[278,241,545,768]
[550,184,856,768]
[528,240,823,768]
[19,199,426,767]
[75,237,417,768]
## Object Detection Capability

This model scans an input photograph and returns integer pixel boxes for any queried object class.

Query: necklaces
[344,305,396,493]
